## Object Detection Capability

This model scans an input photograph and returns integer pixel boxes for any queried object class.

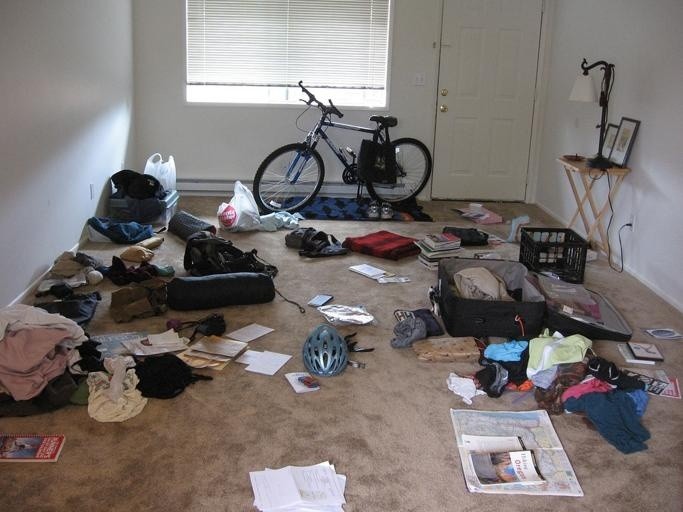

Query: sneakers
[380,202,397,219]
[365,199,380,218]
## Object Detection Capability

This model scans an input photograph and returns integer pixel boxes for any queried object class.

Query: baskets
[519,227,587,284]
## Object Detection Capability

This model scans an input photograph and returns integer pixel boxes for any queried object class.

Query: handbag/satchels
[356,131,396,184]
[443,227,488,246]
[135,355,192,398]
[285,227,350,258]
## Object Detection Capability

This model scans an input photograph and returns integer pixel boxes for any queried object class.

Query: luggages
[429,256,633,341]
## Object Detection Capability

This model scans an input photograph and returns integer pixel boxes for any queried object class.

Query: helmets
[303,323,349,377]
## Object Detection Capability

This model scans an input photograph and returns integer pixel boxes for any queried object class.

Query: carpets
[282,197,432,221]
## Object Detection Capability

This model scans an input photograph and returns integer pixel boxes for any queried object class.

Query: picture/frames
[601,117,641,169]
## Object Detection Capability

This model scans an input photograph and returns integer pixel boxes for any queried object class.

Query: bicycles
[253,81,432,213]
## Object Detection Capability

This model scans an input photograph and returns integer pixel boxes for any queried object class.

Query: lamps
[567,58,615,169]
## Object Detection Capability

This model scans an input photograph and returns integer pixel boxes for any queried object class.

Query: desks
[556,157,631,265]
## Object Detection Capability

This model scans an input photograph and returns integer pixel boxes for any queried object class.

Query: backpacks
[184,231,278,279]
[110,170,165,199]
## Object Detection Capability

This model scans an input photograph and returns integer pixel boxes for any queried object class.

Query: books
[617,341,665,366]
[0,436,66,463]
[414,233,464,273]
[183,335,250,363]
[464,435,549,488]
